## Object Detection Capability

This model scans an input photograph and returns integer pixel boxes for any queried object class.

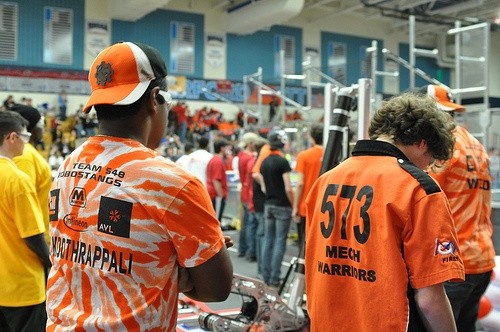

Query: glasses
[7,132,31,142]
[146,89,172,110]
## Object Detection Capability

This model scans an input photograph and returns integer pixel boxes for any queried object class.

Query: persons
[176,126,324,287]
[2,94,316,188]
[416,84,495,331]
[3,103,53,249]
[304,93,466,332]
[1,109,52,332]
[46,41,233,332]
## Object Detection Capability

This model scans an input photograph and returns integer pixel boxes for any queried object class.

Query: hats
[237,133,258,147]
[84,40,169,114]
[10,104,41,132]
[420,83,465,113]
